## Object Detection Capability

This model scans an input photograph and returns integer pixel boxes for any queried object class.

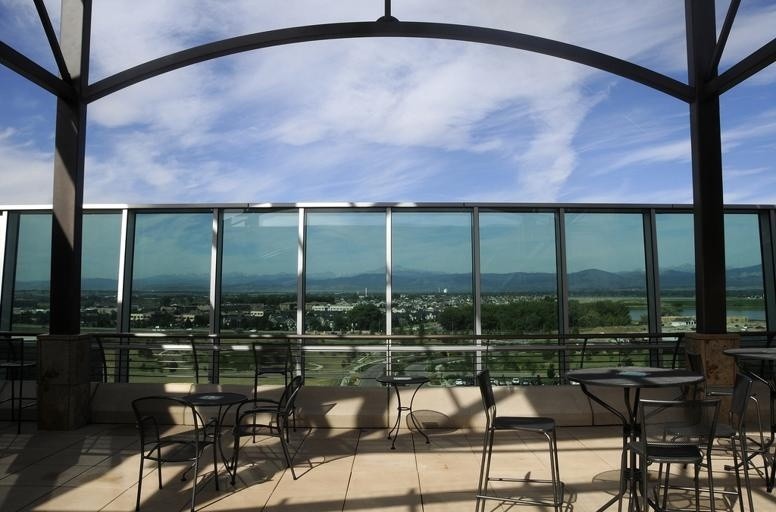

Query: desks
[181,393,248,491]
[722,347,776,492]
[376,376,431,450]
[568,366,706,511]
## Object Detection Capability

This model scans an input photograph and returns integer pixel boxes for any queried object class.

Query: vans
[512,377,520,385]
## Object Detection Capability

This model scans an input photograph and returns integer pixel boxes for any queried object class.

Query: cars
[489,379,495,384]
[522,379,529,385]
[455,379,463,386]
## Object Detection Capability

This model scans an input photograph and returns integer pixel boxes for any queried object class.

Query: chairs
[629,397,721,511]
[476,368,566,511]
[0,338,37,434]
[230,375,305,485]
[657,373,756,512]
[686,350,764,448]
[132,396,218,512]
